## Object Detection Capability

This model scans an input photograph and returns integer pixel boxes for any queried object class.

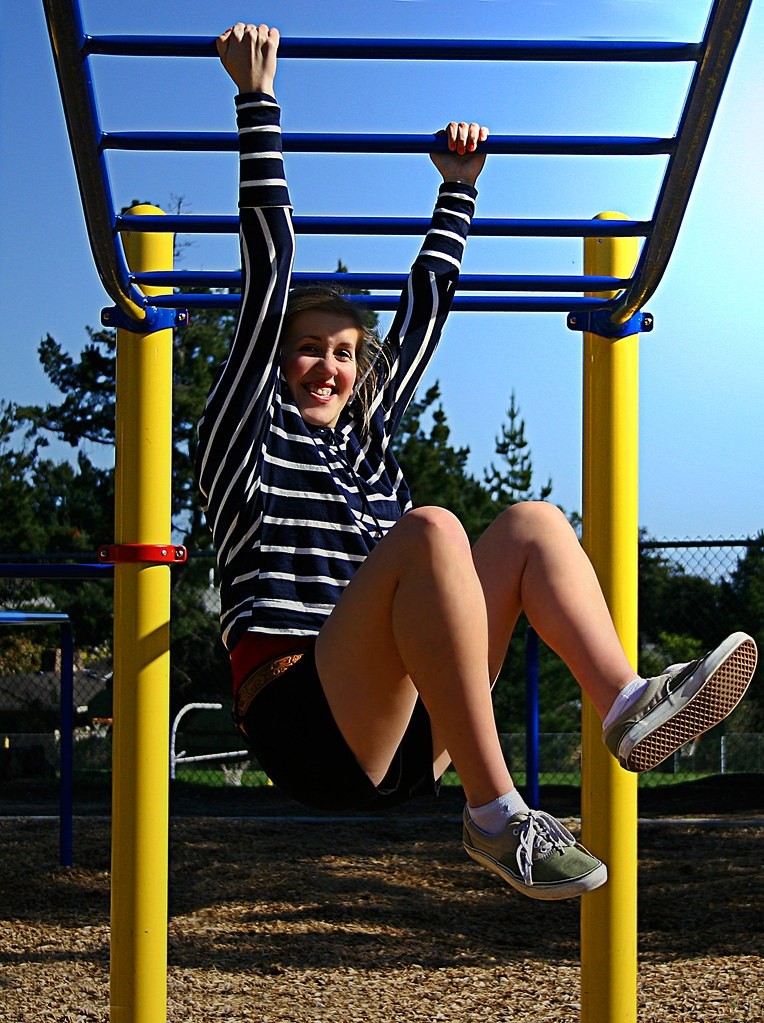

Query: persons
[195,22,757,899]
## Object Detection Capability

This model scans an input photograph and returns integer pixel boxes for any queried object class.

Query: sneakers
[461,802,608,900]
[601,631,758,775]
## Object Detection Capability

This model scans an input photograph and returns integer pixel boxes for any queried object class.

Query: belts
[231,653,305,738]
